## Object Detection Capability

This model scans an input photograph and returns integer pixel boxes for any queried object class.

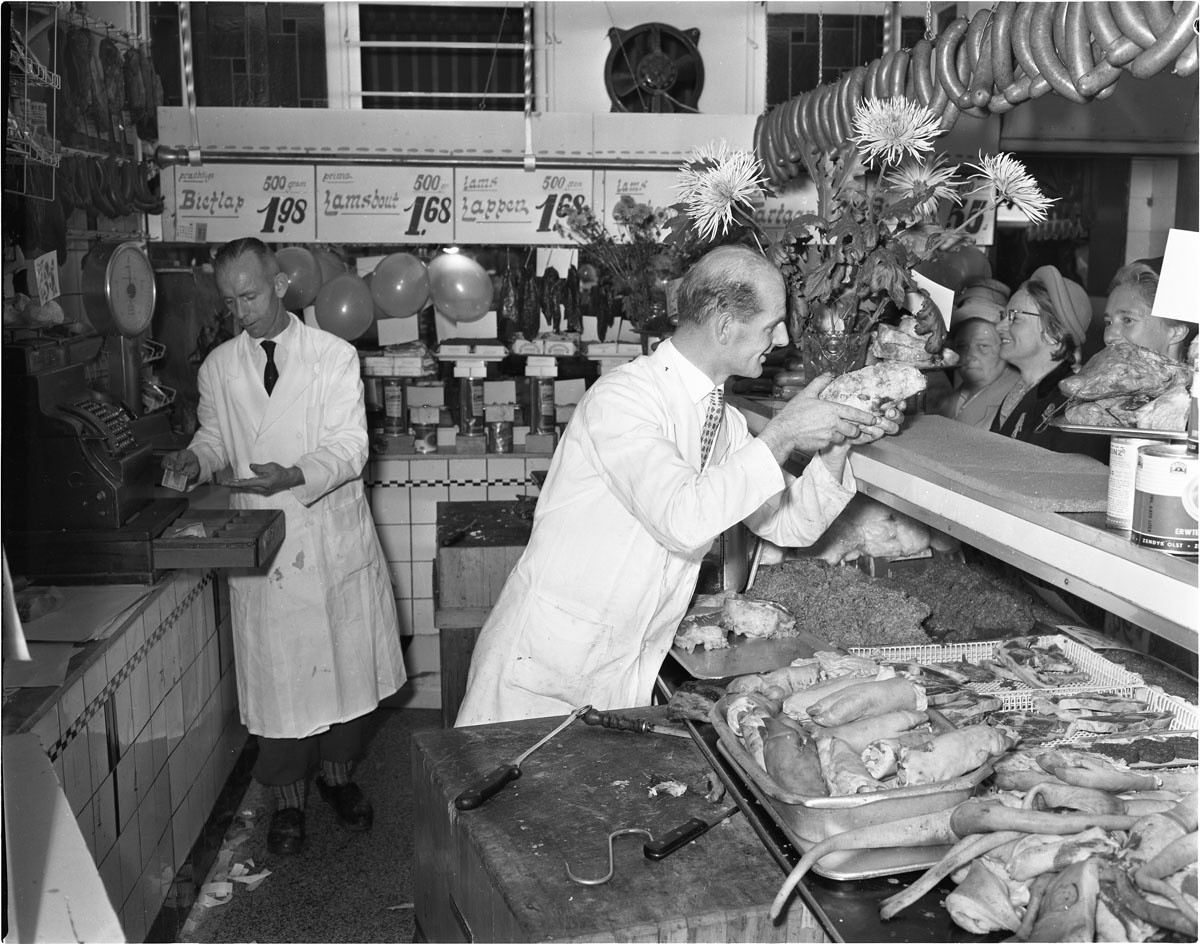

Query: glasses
[999,309,1040,321]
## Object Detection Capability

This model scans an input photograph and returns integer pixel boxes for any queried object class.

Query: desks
[409,708,844,944]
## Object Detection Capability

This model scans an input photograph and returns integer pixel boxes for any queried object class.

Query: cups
[489,420,513,453]
[414,423,437,453]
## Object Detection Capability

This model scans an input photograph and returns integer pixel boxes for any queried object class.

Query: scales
[84,239,196,450]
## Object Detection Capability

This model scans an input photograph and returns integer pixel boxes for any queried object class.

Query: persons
[1102,253,1199,365]
[939,295,1022,431]
[986,264,1111,460]
[153,235,409,859]
[447,240,908,731]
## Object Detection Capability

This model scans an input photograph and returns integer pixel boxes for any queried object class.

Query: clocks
[606,22,706,113]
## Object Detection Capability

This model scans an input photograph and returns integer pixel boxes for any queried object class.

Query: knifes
[441,518,478,546]
[642,798,748,861]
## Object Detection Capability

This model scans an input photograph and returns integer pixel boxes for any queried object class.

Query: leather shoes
[314,774,374,832]
[267,806,306,854]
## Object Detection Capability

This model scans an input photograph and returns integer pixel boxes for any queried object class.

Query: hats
[1029,265,1093,348]
[948,297,1004,328]
[953,276,1011,308]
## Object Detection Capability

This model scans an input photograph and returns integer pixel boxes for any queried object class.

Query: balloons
[270,244,495,340]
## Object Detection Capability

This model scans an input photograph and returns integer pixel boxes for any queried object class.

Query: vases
[675,90,1066,398]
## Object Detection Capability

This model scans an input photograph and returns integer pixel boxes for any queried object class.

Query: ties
[700,389,725,474]
[260,341,279,397]
